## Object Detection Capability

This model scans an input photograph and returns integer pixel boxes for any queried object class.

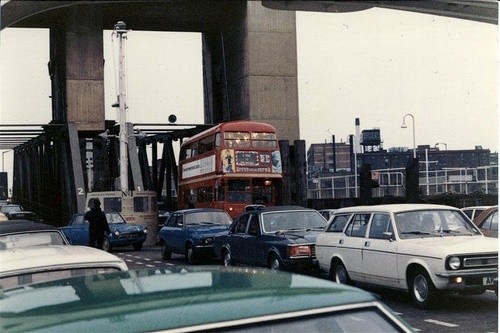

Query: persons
[84,200,112,249]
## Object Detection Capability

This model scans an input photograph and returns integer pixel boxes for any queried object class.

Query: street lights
[400,113,417,159]
[111,19,129,192]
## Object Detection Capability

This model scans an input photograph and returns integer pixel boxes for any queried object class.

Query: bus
[177,121,285,220]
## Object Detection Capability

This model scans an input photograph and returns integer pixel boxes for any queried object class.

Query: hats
[93,199,100,206]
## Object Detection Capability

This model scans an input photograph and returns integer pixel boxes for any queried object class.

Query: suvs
[316,203,498,308]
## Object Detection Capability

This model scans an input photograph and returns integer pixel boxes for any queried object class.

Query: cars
[222,205,331,271]
[475,207,499,238]
[0,244,129,293]
[60,209,149,253]
[0,218,70,251]
[0,204,36,220]
[158,208,233,262]
[0,263,423,332]
[451,206,490,227]
